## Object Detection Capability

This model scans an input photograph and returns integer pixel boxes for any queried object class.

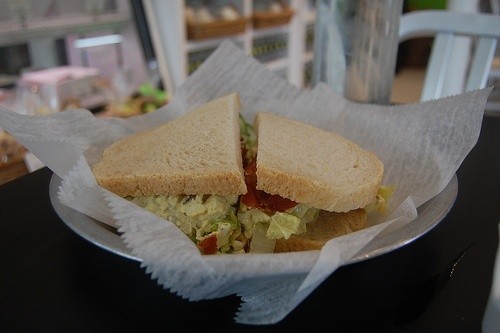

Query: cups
[312,1,402,106]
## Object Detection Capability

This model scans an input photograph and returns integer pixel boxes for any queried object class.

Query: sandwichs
[92,93,396,254]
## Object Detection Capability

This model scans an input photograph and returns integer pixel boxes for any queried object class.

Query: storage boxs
[23,65,100,111]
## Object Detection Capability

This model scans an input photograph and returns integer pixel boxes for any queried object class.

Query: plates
[49,172,458,275]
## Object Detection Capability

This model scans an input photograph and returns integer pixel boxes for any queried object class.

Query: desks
[0,117,500,333]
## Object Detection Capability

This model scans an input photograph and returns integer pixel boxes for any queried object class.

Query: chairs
[398,13,500,113]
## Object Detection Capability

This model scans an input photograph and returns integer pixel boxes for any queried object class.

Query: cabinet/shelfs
[153,0,318,104]
